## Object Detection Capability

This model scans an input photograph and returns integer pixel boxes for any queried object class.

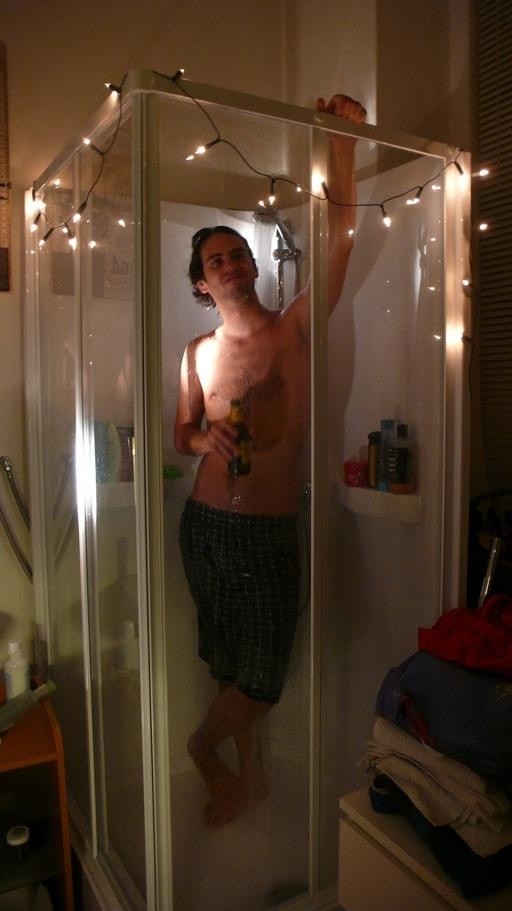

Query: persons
[171,95,366,829]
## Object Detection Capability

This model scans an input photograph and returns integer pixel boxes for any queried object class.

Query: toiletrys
[3,640,32,702]
[116,619,139,670]
[342,417,415,495]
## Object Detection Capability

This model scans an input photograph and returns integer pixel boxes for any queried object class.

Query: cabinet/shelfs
[1,676,76,910]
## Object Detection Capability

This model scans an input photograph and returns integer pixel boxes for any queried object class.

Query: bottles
[225,397,252,477]
[368,419,415,494]
